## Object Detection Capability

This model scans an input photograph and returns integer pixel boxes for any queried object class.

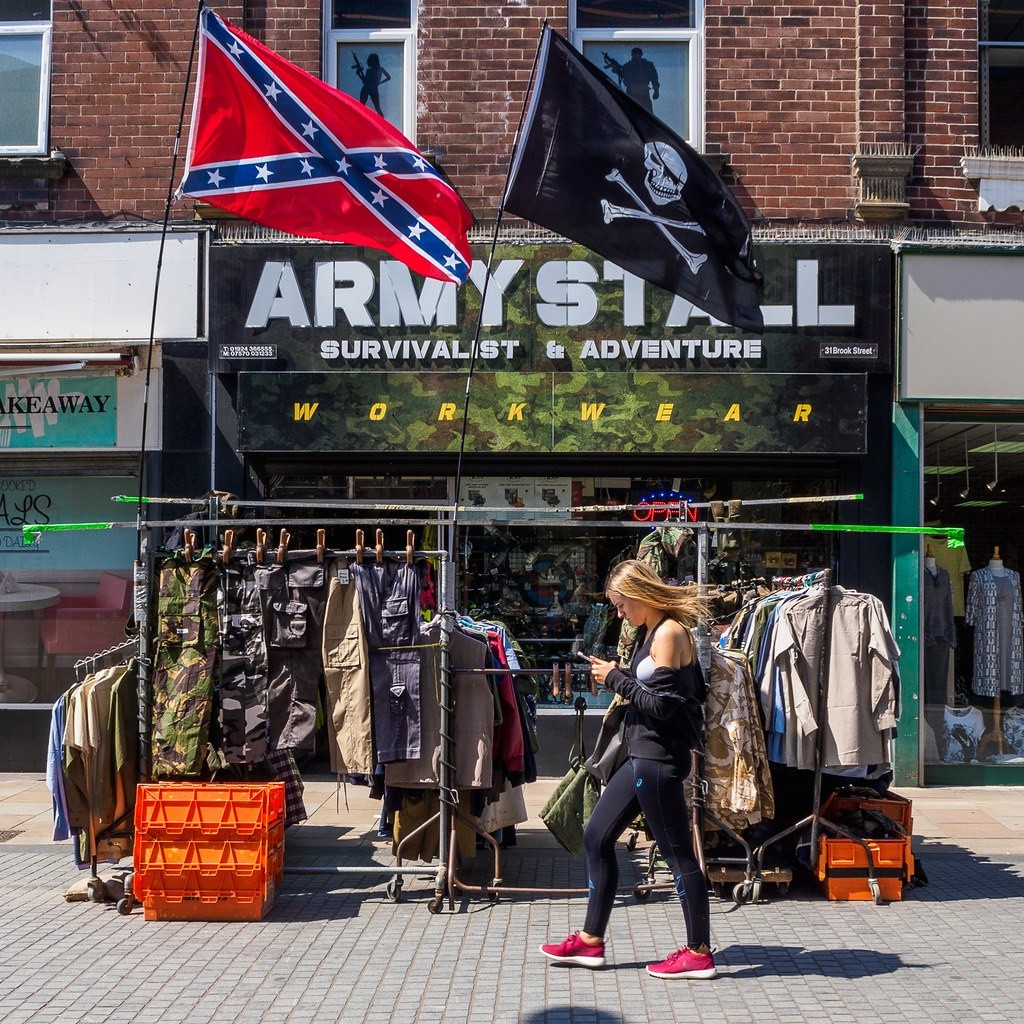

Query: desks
[0,583,61,703]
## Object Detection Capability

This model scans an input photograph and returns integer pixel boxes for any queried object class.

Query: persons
[540,559,719,981]
[965,560,1024,697]
[573,583,604,615]
[923,556,956,702]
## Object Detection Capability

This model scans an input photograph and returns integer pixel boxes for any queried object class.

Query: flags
[503,27,763,334]
[177,9,476,292]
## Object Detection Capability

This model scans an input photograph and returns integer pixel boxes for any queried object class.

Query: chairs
[36,572,133,703]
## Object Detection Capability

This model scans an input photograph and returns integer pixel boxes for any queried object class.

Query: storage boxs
[814,834,914,901]
[132,780,287,922]
[819,790,914,835]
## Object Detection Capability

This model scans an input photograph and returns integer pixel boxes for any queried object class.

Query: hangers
[769,570,825,592]
[75,640,140,683]
[930,522,946,539]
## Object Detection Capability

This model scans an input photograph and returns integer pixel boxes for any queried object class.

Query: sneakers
[645,945,717,979]
[539,931,606,967]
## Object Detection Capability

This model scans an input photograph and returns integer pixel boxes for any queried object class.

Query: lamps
[959,432,970,499]
[986,424,998,491]
[930,441,940,506]
